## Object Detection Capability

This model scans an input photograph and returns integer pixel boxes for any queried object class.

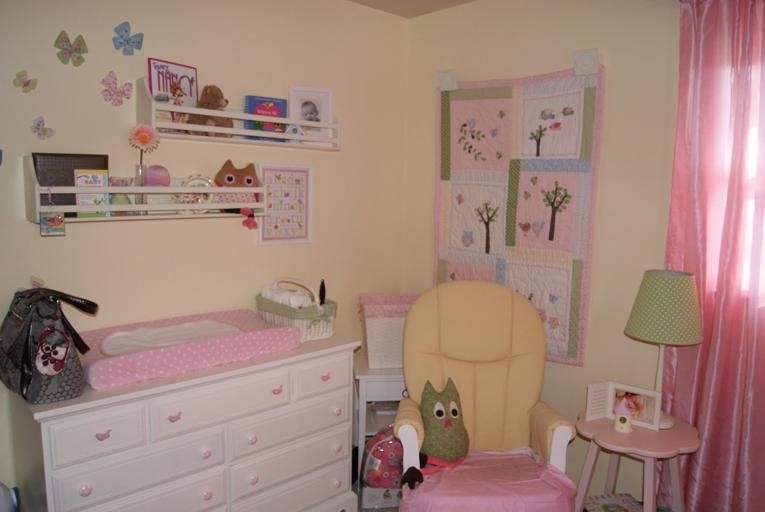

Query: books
[73,168,110,219]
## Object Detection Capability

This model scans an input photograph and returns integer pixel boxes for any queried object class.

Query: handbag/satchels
[0,286,101,406]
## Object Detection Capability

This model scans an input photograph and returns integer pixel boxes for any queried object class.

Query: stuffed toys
[416,377,470,466]
[184,85,233,139]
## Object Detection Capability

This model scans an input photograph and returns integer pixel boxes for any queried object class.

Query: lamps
[623,269,702,430]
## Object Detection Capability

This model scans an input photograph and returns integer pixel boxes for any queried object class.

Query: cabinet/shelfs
[12,329,406,512]
[22,154,269,224]
[135,79,340,152]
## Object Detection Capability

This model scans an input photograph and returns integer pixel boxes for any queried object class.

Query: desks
[574,407,700,511]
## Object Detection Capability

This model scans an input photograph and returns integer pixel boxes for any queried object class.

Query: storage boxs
[256,287,339,342]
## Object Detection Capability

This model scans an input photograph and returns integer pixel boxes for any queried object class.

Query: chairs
[394,280,577,512]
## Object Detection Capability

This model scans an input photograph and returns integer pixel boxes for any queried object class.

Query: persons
[300,100,320,131]
[614,391,641,419]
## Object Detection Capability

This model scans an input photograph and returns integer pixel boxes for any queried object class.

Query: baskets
[253,292,340,345]
